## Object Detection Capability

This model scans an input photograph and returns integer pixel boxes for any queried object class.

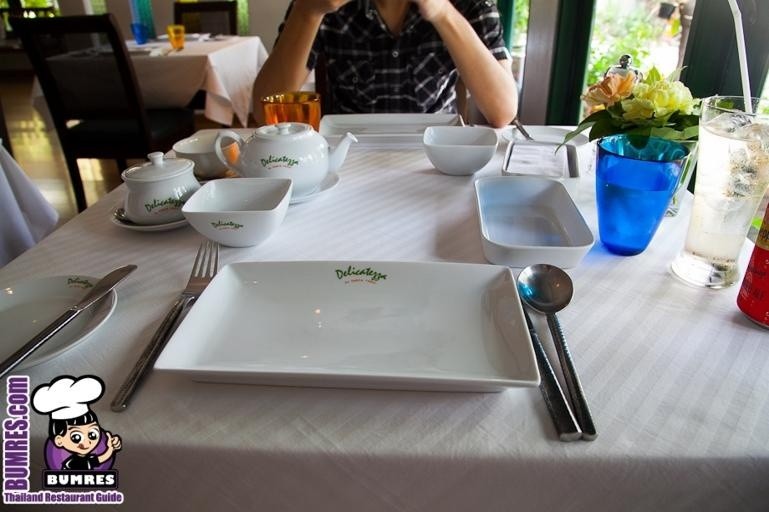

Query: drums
[2,257,142,385]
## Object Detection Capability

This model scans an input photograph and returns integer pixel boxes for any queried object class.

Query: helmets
[151,261,549,395]
[500,123,589,149]
[472,176,595,271]
[4,272,117,375]
[111,204,195,233]
[286,169,341,205]
[318,112,465,146]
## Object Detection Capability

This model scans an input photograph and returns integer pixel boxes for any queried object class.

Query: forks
[113,235,224,412]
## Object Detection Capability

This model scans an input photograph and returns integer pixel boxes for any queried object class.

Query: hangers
[515,260,600,442]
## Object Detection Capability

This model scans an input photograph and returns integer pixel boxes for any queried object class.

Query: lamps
[173,0,243,117]
[307,41,472,120]
[7,8,198,214]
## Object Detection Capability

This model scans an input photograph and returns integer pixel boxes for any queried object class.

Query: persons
[247,0,520,131]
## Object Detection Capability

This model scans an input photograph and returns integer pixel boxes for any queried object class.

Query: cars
[130,22,149,45]
[736,194,769,331]
[667,96,769,290]
[166,24,186,50]
[261,91,321,133]
[117,148,205,223]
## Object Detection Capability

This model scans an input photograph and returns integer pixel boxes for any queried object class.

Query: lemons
[212,122,359,193]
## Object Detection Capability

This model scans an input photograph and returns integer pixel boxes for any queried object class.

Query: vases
[552,66,736,152]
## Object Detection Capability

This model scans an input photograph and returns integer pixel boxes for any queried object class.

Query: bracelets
[422,122,497,177]
[180,175,297,249]
[171,131,233,173]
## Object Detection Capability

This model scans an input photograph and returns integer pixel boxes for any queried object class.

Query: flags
[664,138,699,218]
